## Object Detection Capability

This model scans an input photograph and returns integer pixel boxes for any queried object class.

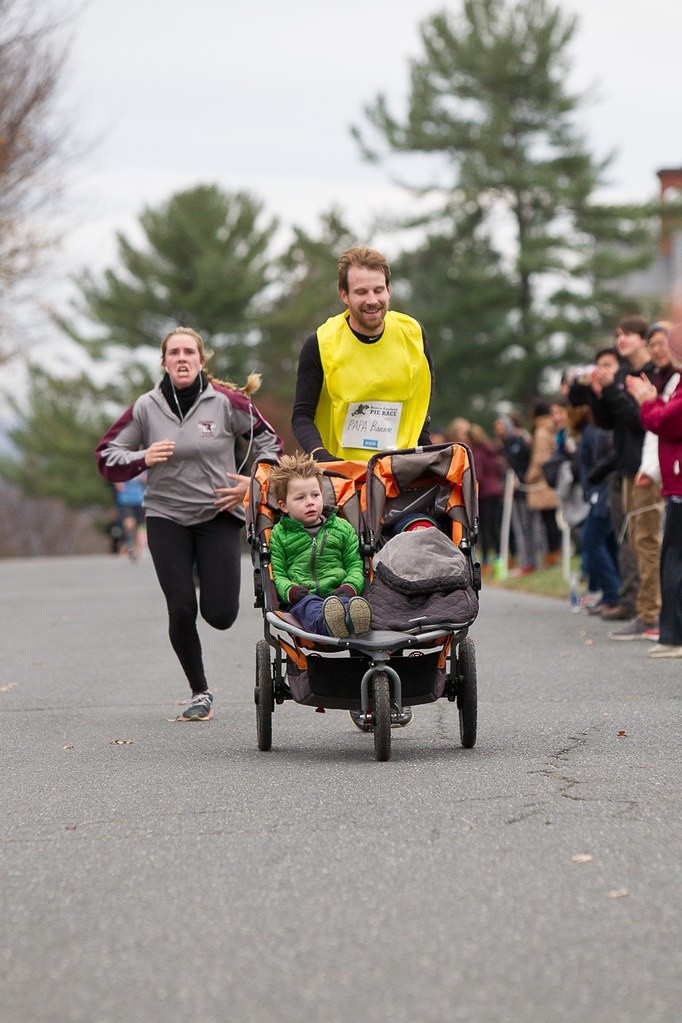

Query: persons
[97,328,285,722]
[270,451,372,639]
[430,313,682,658]
[392,512,455,628]
[293,249,435,733]
[113,469,149,563]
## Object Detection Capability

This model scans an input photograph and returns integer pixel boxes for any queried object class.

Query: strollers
[243,442,479,761]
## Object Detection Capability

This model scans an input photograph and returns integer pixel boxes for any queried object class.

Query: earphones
[164,365,169,374]
[199,363,202,371]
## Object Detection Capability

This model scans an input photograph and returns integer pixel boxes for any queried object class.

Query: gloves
[289,585,308,605]
[336,584,355,597]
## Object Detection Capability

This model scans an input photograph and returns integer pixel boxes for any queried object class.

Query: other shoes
[601,604,629,619]
[588,604,610,614]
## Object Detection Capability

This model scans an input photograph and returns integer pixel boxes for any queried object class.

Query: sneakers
[649,643,682,658]
[349,709,364,730]
[392,706,414,727]
[645,629,661,641]
[609,620,650,639]
[323,595,350,638]
[181,692,213,720]
[347,595,371,633]
